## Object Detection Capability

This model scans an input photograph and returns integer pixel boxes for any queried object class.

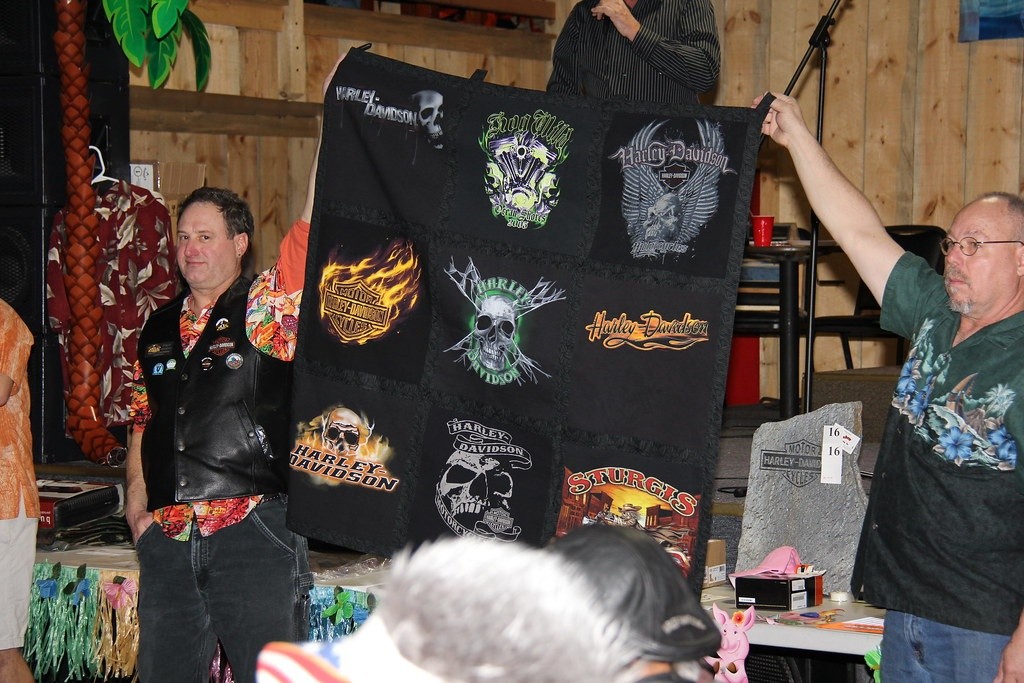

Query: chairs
[812,224,947,370]
[733,225,813,338]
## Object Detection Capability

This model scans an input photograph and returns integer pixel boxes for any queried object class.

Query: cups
[751,216,775,247]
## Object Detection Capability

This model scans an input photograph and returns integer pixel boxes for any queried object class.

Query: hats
[546,523,722,662]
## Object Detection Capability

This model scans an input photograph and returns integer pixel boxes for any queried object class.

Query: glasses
[939,237,1024,256]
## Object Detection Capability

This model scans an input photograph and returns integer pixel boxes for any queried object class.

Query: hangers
[89,145,120,185]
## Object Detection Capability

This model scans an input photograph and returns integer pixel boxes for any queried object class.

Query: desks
[733,245,843,420]
[701,583,885,683]
[21,543,390,683]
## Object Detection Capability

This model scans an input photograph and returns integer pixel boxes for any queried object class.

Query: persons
[253,523,719,683]
[0,297,41,683]
[124,51,350,683]
[545,0,721,105]
[749,92,1024,683]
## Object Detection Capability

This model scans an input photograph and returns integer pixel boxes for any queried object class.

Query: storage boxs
[157,160,208,246]
[735,576,823,612]
[702,539,727,590]
